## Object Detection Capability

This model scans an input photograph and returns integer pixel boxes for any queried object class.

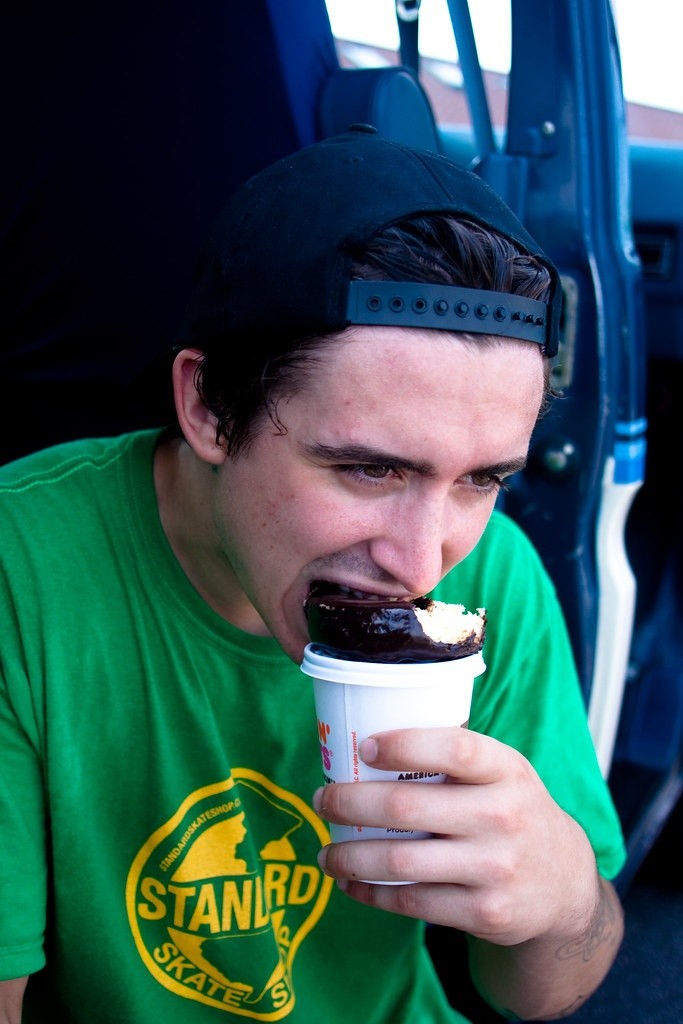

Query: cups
[293,643,486,887]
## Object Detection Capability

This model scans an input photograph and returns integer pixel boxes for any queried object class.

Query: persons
[0,125,626,1024]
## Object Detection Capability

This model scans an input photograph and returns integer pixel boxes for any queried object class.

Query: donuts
[302,584,488,665]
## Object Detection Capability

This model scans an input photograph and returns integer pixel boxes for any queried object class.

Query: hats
[214,123,568,358]
